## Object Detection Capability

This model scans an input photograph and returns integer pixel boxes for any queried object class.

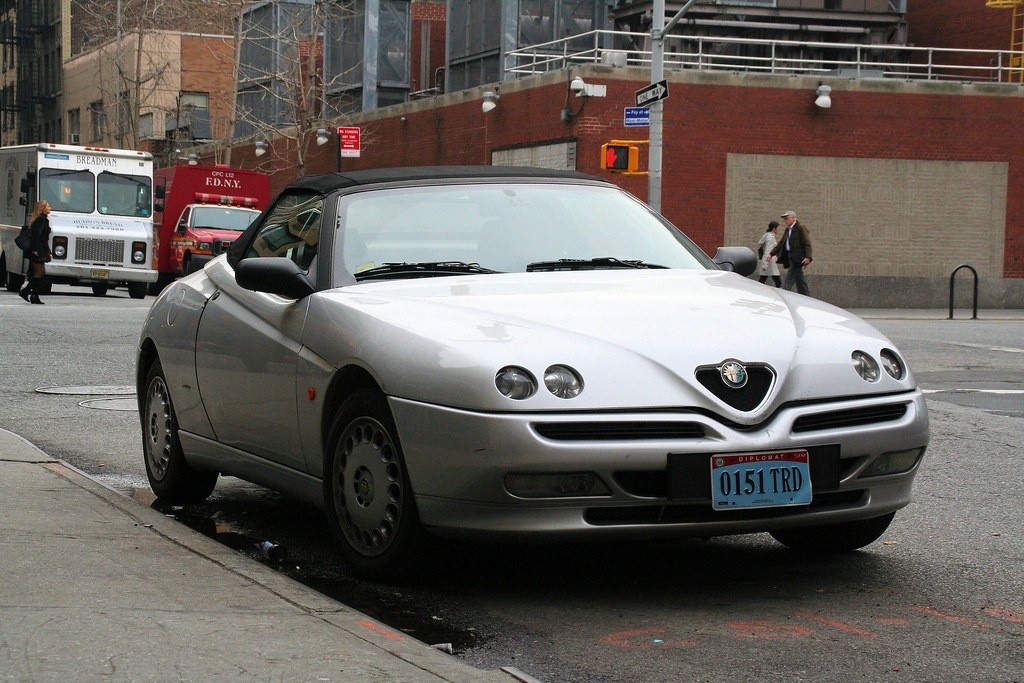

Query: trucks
[0,142,158,298]
[155,166,272,278]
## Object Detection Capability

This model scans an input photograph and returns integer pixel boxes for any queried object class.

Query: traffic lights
[601,138,650,177]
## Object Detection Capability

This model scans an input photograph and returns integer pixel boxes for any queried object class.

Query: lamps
[188,154,201,166]
[255,142,268,157]
[482,91,500,113]
[814,85,831,108]
[316,129,331,146]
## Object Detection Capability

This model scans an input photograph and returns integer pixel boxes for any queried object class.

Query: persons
[757,221,782,289]
[764,211,814,296]
[17,200,52,305]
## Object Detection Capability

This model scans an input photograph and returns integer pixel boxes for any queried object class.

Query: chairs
[344,227,381,275]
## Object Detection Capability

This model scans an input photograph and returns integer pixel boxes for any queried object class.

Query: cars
[134,161,933,581]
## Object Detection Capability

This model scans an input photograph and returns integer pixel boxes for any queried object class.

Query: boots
[19,284,43,304]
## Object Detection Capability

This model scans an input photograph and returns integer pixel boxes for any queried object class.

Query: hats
[781,211,797,218]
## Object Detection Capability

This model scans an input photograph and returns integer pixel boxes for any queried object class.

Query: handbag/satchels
[758,245,764,259]
[15,224,32,253]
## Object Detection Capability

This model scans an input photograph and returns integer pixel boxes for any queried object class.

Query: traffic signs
[634,79,669,108]
[625,107,650,127]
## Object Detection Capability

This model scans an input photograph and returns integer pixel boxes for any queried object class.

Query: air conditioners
[601,52,628,66]
